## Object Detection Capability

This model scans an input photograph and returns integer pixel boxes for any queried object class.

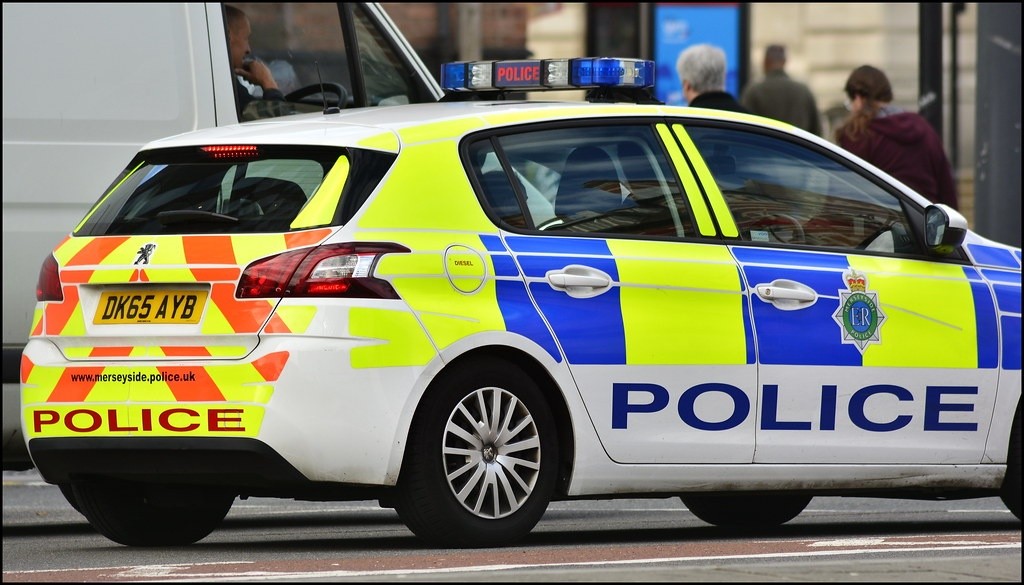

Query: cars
[19,55,1023,547]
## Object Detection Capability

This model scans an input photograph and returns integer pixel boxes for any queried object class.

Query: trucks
[3,3,444,473]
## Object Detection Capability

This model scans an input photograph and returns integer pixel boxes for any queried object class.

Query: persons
[833,65,959,215]
[222,2,290,123]
[739,45,826,136]
[676,44,750,114]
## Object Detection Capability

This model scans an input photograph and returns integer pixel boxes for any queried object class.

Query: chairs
[542,144,636,231]
[228,176,308,233]
